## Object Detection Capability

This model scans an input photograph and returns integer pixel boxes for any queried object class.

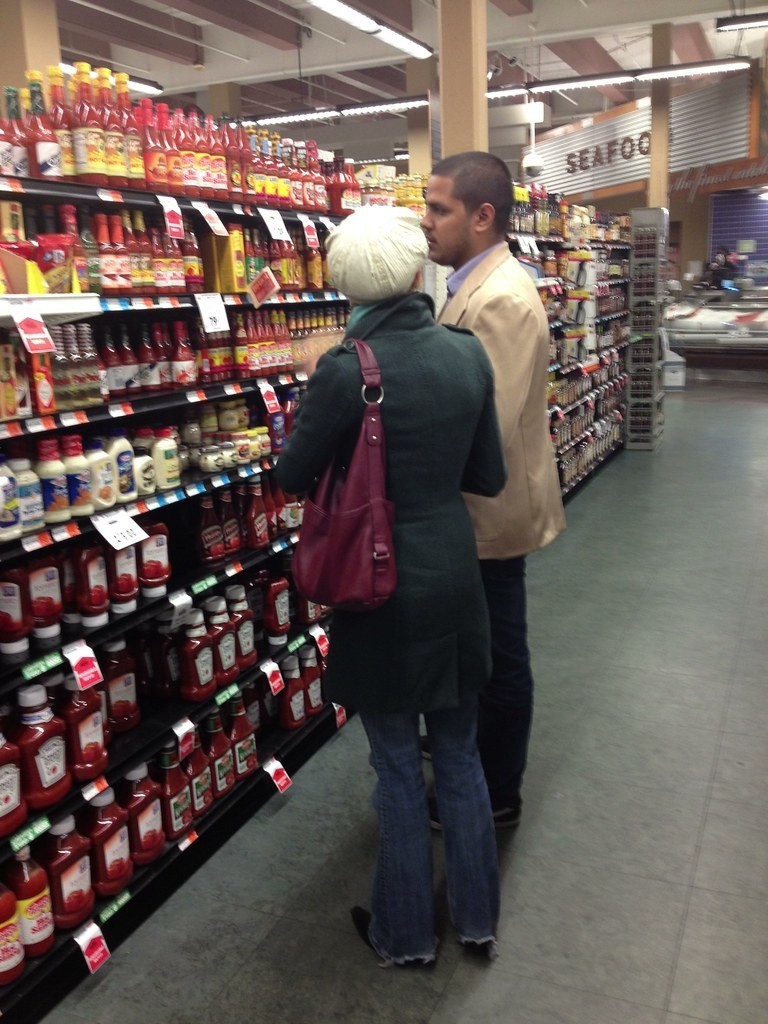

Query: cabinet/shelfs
[0,168,426,1024]
[503,230,632,503]
[627,207,673,453]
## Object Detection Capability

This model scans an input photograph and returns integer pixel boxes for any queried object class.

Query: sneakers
[424,798,524,831]
[419,734,434,760]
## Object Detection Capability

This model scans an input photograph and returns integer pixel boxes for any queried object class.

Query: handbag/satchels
[289,402,395,614]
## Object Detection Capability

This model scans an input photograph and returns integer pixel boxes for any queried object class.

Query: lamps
[485,0,768,101]
[58,0,434,129]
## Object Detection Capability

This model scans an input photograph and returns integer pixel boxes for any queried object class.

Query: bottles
[508,249,632,487]
[22,200,206,293]
[0,61,429,214]
[0,520,171,654]
[243,225,331,291]
[141,546,345,701]
[46,304,351,411]
[0,639,140,839]
[0,618,347,989]
[199,469,307,561]
[0,384,311,543]
[507,180,631,245]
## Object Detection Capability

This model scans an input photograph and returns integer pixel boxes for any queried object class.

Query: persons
[275,201,505,964]
[418,151,567,837]
[703,245,738,288]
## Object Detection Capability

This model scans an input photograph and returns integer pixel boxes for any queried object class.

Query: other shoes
[459,939,493,962]
[349,905,439,972]
